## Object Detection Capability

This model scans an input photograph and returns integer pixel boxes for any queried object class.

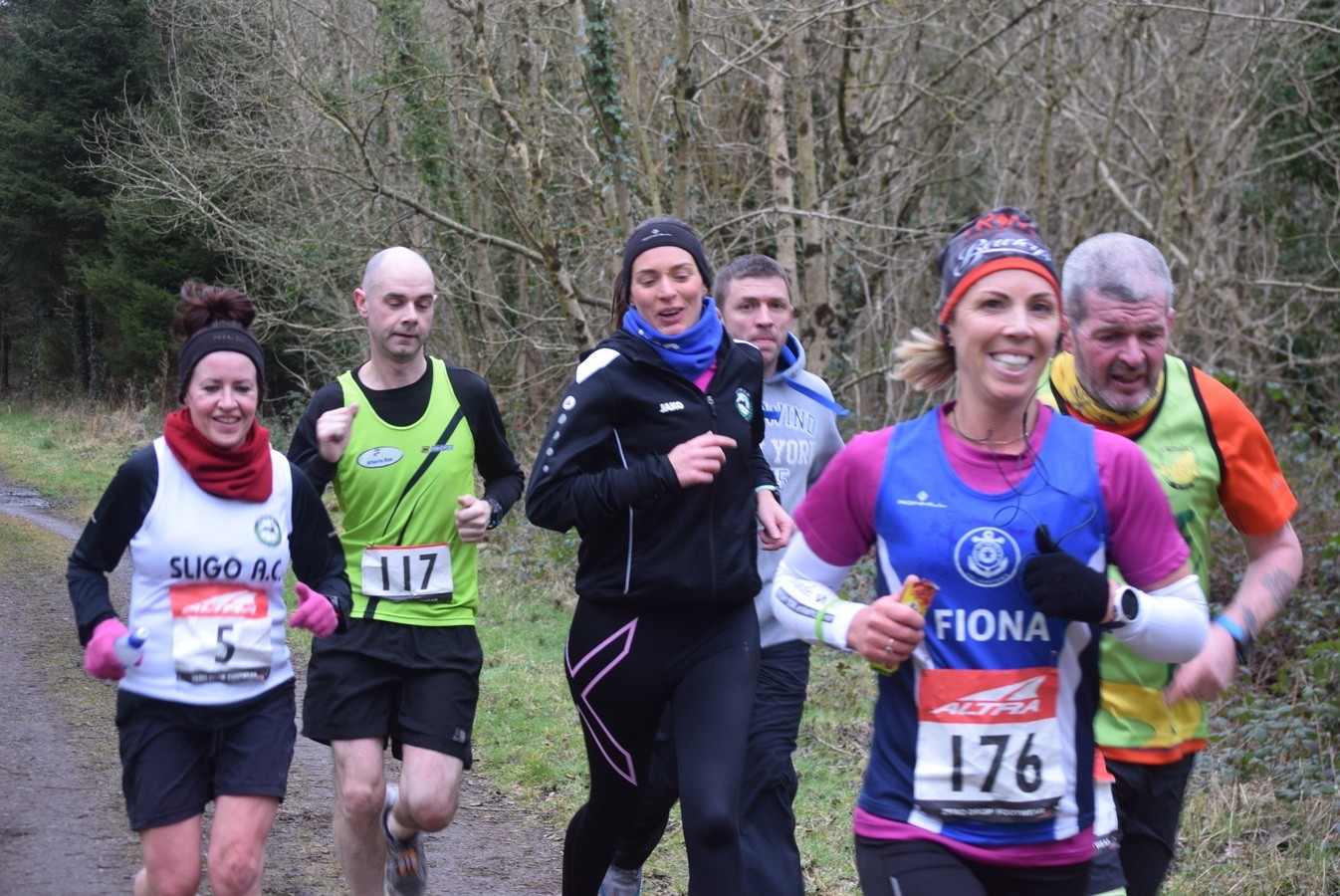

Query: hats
[933,205,1064,326]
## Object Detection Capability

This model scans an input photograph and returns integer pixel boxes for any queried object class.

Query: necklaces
[944,414,1030,446]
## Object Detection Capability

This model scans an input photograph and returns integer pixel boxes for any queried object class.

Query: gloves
[291,581,337,638]
[1022,524,1108,624]
[84,617,143,681]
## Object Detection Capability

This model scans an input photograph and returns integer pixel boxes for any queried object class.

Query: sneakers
[598,865,642,896]
[379,783,428,896]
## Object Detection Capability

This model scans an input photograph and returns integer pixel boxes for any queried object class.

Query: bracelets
[815,596,841,653]
[1213,614,1245,654]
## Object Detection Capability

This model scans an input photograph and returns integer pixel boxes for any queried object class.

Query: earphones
[1056,333,1064,350]
[939,325,949,334]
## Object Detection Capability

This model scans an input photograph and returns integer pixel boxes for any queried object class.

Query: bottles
[99,626,150,686]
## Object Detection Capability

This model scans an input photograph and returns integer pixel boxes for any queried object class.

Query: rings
[885,638,894,654]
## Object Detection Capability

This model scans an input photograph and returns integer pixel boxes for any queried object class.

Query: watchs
[1096,582,1143,633]
[484,497,504,531]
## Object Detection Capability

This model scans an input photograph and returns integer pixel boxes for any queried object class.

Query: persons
[1036,233,1303,895]
[62,279,352,893]
[700,245,850,895]
[288,242,525,894]
[770,205,1214,895]
[523,217,800,895]
